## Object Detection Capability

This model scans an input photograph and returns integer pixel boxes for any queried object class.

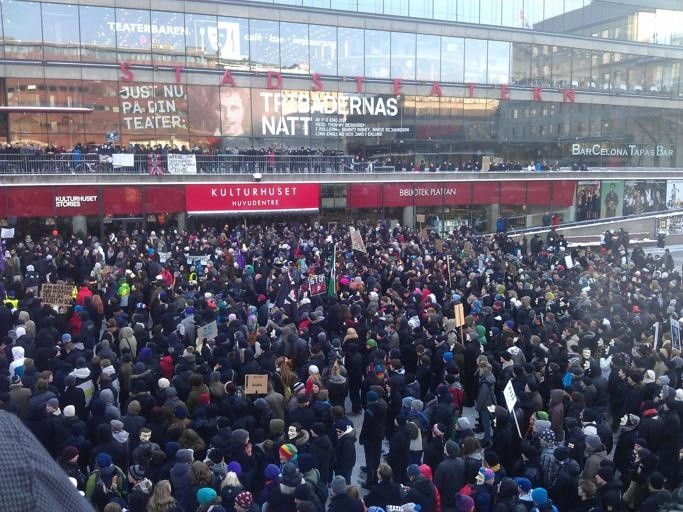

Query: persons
[0,139,683,511]
[206,86,254,147]
[104,126,120,146]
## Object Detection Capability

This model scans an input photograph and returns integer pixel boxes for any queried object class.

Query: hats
[577,479,597,496]
[648,471,664,491]
[0,301,679,481]
[500,477,517,495]
[234,490,254,509]
[196,487,218,506]
[401,502,422,512]
[514,476,532,492]
[454,493,474,511]
[330,475,347,493]
[292,483,311,501]
[531,488,548,504]
